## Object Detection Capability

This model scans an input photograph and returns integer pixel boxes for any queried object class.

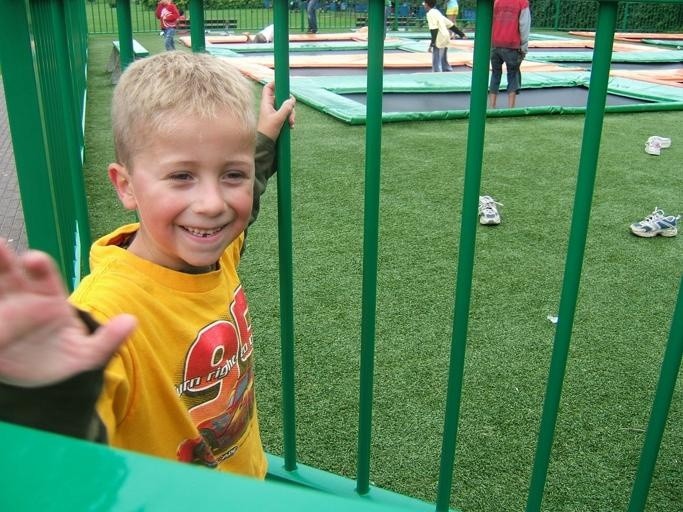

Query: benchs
[106,38,150,85]
[176,19,238,35]
[356,17,417,32]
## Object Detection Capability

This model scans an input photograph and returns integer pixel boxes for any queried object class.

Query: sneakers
[477,193,501,226]
[628,204,679,238]
[644,136,671,156]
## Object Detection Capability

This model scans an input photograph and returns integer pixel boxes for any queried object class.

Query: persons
[445,1,459,40]
[423,1,465,73]
[304,0,319,34]
[0,50,299,483]
[153,0,180,50]
[487,0,531,109]
